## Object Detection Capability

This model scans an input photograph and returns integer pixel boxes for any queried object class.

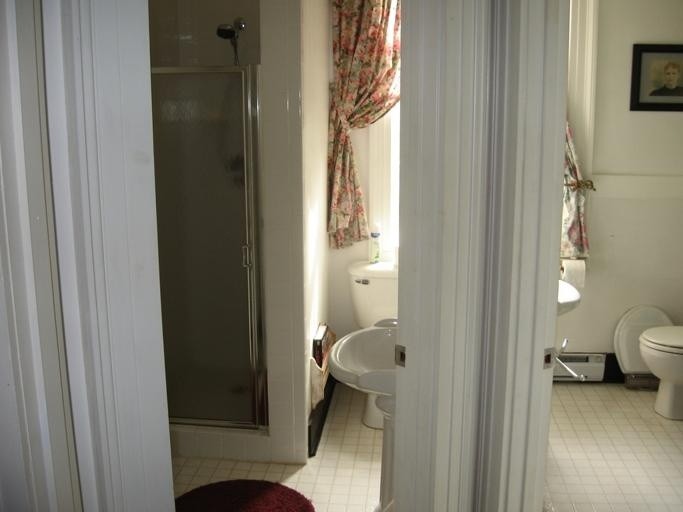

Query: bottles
[367,232,380,264]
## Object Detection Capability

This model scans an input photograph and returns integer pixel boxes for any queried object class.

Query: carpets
[176,479,317,511]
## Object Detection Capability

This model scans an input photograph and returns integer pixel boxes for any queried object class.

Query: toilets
[327,260,398,430]
[637,326,683,422]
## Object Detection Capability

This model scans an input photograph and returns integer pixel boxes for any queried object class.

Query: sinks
[558,279,581,313]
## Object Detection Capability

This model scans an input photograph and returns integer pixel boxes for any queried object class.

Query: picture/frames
[630,45,683,110]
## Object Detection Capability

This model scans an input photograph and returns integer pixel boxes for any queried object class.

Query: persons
[649,61,683,96]
